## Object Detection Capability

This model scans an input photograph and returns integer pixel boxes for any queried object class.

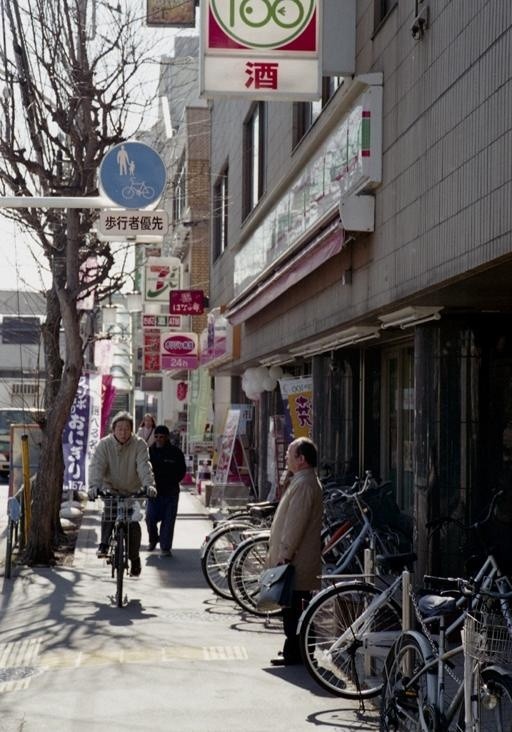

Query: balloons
[240,362,296,401]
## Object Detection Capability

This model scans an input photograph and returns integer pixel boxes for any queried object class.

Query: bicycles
[294,487,512,715]
[89,485,156,606]
[200,469,393,624]
[381,573,512,731]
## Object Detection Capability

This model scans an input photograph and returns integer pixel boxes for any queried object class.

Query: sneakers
[148,533,160,550]
[96,543,110,558]
[269,652,307,667]
[127,557,142,577]
[159,549,171,557]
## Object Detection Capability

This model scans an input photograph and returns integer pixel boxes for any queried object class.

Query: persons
[86,409,159,579]
[135,412,158,447]
[143,424,188,558]
[261,434,326,669]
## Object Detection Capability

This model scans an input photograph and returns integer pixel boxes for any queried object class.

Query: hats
[154,425,169,437]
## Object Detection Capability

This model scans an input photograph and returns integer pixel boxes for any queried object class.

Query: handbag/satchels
[254,560,297,608]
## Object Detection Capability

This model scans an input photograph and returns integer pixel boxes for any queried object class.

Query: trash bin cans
[1,461,11,484]
